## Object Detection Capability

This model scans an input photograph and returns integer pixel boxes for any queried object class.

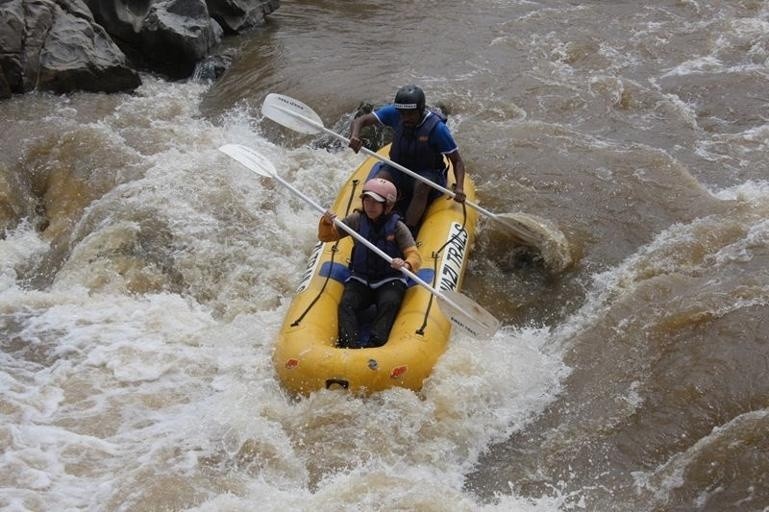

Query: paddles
[218,143,500,342]
[262,93,549,246]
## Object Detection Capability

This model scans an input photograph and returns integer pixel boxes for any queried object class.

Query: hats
[360,191,386,203]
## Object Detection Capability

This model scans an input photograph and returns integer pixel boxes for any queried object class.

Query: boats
[270,142,479,407]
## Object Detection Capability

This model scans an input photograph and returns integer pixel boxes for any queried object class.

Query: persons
[348,82,466,236]
[318,177,424,348]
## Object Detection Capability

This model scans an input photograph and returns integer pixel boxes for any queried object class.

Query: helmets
[363,178,397,215]
[395,86,425,115]
